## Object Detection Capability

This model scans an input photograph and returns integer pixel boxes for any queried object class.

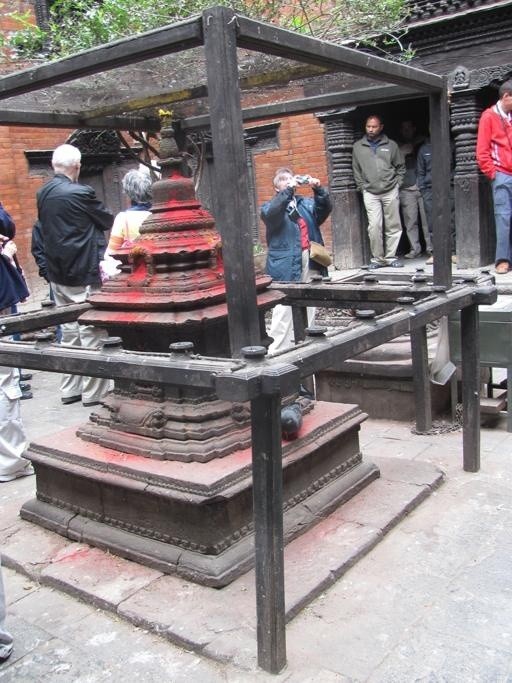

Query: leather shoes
[20,373,103,407]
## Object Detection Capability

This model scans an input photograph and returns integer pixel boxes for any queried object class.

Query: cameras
[297,175,309,184]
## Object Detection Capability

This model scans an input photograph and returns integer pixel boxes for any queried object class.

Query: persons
[1,204,34,379]
[260,165,334,353]
[475,79,511,273]
[103,167,154,277]
[395,113,433,257]
[31,218,63,343]
[37,143,114,406]
[350,114,405,268]
[1,559,15,660]
[417,124,457,264]
[0,254,37,482]
[0,234,33,401]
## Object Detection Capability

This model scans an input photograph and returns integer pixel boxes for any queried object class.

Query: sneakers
[368,261,381,268]
[0,461,36,482]
[404,251,456,264]
[388,260,404,267]
[496,261,509,274]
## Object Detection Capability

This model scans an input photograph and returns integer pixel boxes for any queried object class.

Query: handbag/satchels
[309,240,333,267]
